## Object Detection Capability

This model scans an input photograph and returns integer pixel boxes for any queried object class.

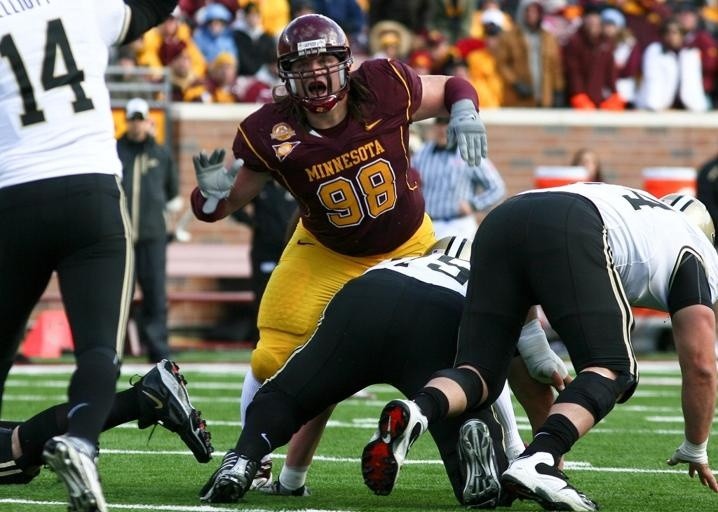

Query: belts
[429,213,466,224]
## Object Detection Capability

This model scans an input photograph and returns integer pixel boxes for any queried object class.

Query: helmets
[277,14,352,112]
[660,193,715,249]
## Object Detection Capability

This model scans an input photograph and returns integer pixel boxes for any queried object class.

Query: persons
[404,115,508,249]
[292,0,718,113]
[570,147,604,188]
[115,92,183,368]
[190,12,491,502]
[197,236,566,510]
[0,0,178,511]
[228,176,299,349]
[109,0,292,102]
[358,176,718,512]
[0,357,215,490]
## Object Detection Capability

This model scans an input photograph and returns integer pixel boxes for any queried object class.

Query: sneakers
[200,451,257,501]
[360,399,426,498]
[43,437,108,512]
[249,452,272,491]
[501,452,599,511]
[138,357,213,463]
[455,417,502,510]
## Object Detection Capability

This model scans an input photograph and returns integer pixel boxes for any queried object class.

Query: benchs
[39,243,253,302]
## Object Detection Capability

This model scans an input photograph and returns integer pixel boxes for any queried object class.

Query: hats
[125,97,148,120]
[599,8,626,30]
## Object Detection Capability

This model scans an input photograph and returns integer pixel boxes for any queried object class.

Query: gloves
[260,481,311,497]
[192,147,245,201]
[445,99,489,168]
